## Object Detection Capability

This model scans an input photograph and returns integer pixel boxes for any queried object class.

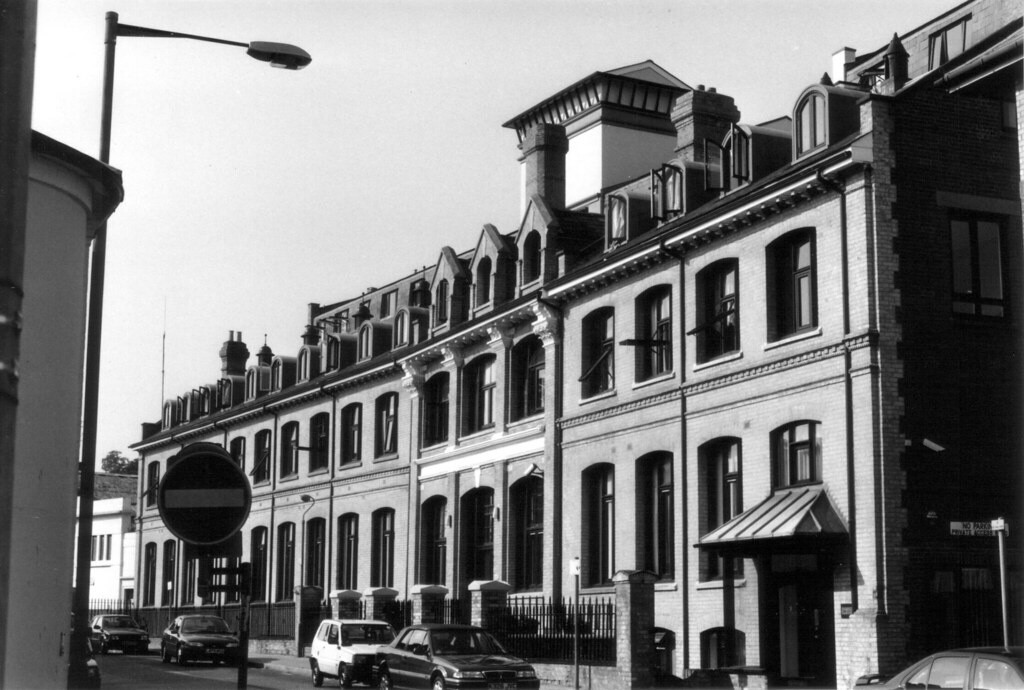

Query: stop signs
[156,449,255,547]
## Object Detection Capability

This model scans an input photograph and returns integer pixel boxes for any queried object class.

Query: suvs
[308,618,397,690]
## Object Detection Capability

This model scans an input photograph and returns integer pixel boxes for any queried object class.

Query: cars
[159,613,239,666]
[853,645,1024,690]
[86,614,152,655]
[372,623,541,690]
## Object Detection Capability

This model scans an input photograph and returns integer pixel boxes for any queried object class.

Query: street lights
[299,493,315,586]
[66,10,316,690]
